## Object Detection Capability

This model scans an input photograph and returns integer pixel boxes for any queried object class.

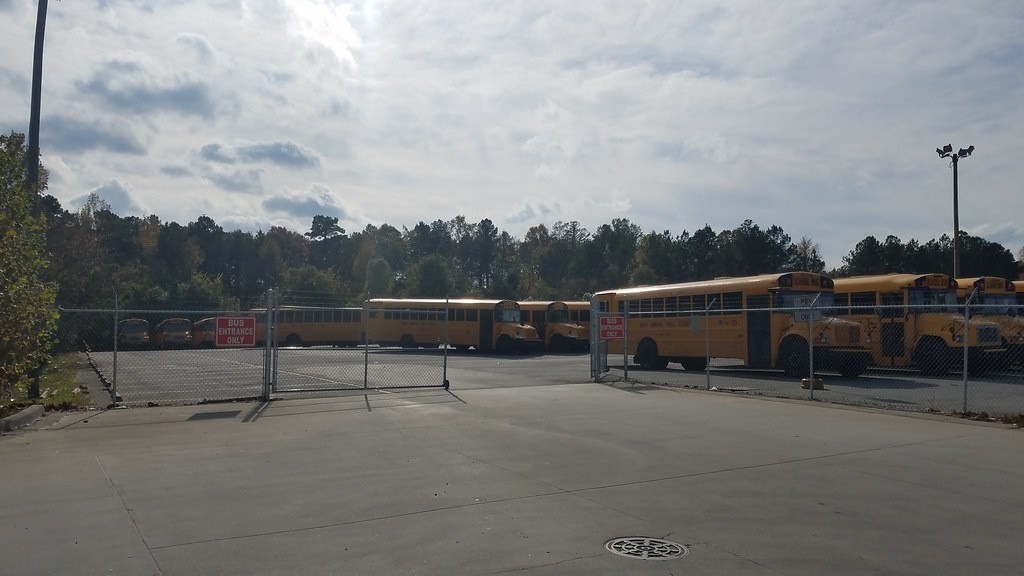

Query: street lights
[936,141,976,280]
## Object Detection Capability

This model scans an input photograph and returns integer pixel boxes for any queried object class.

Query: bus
[362,297,544,356]
[118,317,152,351]
[597,272,873,381]
[154,318,193,350]
[829,273,1007,378]
[952,276,1024,372]
[518,302,587,355]
[566,302,590,337]
[194,315,241,344]
[1011,281,1024,315]
[249,304,365,348]
